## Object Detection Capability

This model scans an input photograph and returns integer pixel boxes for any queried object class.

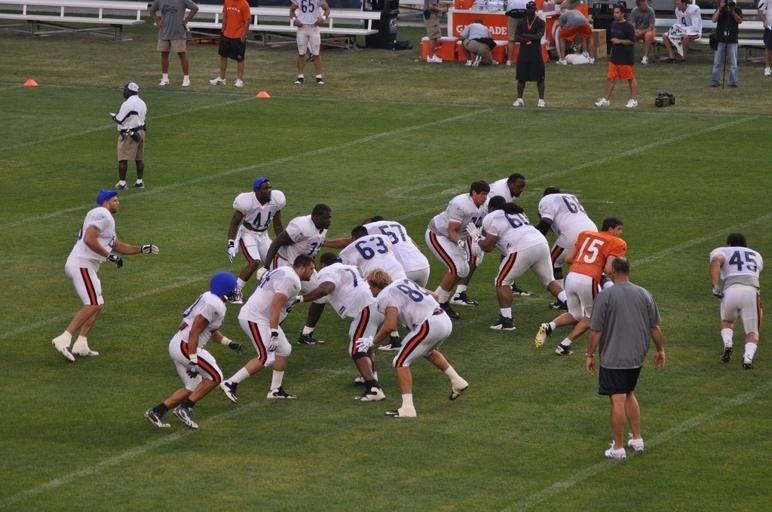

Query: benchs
[638,8,765,64]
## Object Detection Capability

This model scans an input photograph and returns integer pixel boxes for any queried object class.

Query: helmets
[210,272,240,301]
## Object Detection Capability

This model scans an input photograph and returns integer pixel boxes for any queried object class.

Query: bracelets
[292,17,297,21]
[322,16,327,20]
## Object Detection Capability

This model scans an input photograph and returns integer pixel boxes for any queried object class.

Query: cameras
[725,0,736,8]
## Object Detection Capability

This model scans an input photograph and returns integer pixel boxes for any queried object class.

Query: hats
[119,82,139,95]
[97,190,118,205]
[526,1,536,10]
[253,176,269,192]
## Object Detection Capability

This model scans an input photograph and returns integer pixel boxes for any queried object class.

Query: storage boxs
[421,36,549,66]
[586,29,608,58]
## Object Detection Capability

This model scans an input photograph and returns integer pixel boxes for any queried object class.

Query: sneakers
[218,380,240,404]
[51,337,99,363]
[624,98,638,108]
[181,79,191,87]
[232,78,244,88]
[266,386,297,399]
[489,314,517,331]
[173,404,198,429]
[316,78,324,85]
[112,181,144,191]
[297,330,325,345]
[763,67,772,76]
[439,290,479,319]
[663,58,688,64]
[511,98,525,107]
[505,60,511,66]
[556,58,567,66]
[384,408,417,418]
[594,96,611,107]
[538,98,546,108]
[640,55,648,65]
[511,284,532,297]
[465,55,483,68]
[535,297,574,357]
[292,78,304,85]
[377,335,402,351]
[449,377,469,401]
[427,53,443,64]
[158,79,169,85]
[721,343,752,369]
[209,76,227,86]
[354,370,386,402]
[589,58,595,64]
[144,406,171,429]
[604,432,644,461]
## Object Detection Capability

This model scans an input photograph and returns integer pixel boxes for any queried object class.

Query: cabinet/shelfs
[446,6,575,59]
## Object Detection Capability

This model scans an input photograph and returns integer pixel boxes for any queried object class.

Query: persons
[708,233,763,370]
[426,173,598,331]
[506,0,546,107]
[289,0,330,86]
[227,177,286,304]
[144,272,249,430]
[217,203,468,418]
[663,0,702,63]
[594,0,656,107]
[207,1,252,88]
[52,189,160,366]
[758,1,772,75]
[711,1,743,87]
[581,256,666,462]
[110,82,148,191]
[424,0,442,61]
[461,19,493,66]
[148,0,200,87]
[556,10,593,65]
[533,217,626,355]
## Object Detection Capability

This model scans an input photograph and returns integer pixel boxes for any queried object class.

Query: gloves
[186,361,200,378]
[354,336,374,351]
[139,244,159,256]
[711,284,724,299]
[106,253,123,268]
[457,221,486,256]
[256,266,269,281]
[287,295,300,312]
[228,341,248,355]
[226,238,235,264]
[267,328,279,351]
